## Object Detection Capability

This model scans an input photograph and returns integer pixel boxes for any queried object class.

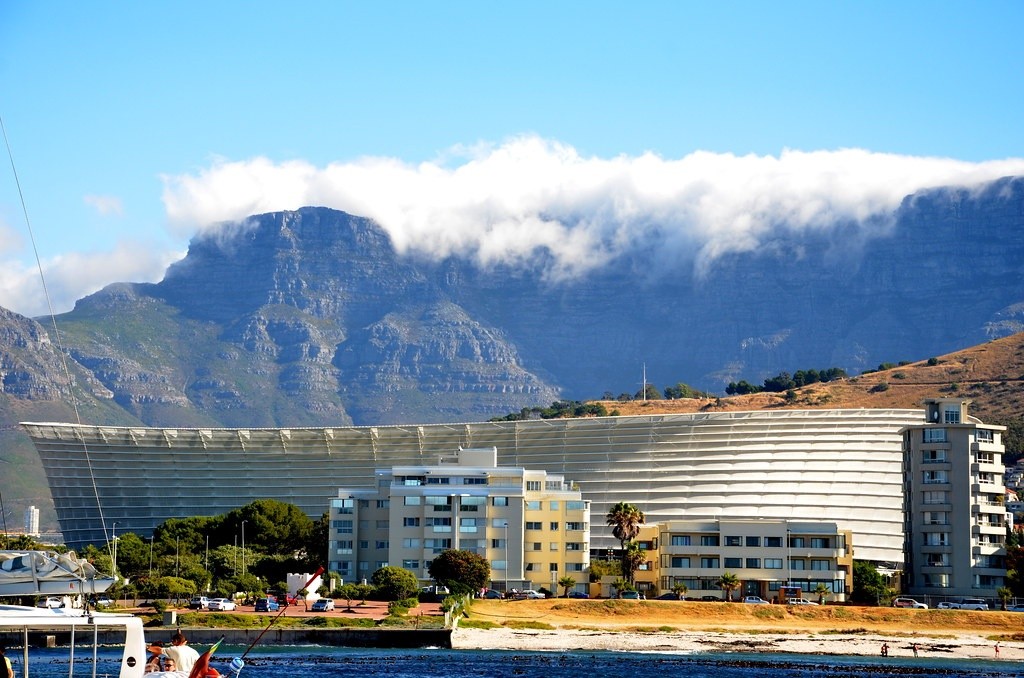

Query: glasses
[164,664,173,667]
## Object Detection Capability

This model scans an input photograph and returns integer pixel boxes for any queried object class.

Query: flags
[190,638,225,678]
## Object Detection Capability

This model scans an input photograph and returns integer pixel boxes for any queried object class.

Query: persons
[146,634,201,673]
[679,588,686,600]
[474,586,488,599]
[511,588,519,594]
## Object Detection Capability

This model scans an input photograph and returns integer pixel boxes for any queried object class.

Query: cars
[208,597,238,612]
[476,589,506,599]
[789,597,819,605]
[744,596,770,604]
[1005,603,1024,613]
[37,596,65,608]
[312,598,334,612]
[610,590,721,602]
[566,591,590,599]
[936,601,962,609]
[132,602,160,617]
[520,590,546,599]
[88,595,115,609]
[421,584,450,595]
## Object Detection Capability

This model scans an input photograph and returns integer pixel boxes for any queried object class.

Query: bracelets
[147,645,149,649]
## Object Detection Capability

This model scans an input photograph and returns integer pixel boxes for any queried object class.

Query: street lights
[150,536,154,577]
[241,520,249,574]
[112,522,116,578]
[176,536,180,576]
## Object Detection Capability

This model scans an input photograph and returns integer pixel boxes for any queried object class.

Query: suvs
[894,597,928,611]
[948,597,991,612]
[190,596,211,610]
[278,594,299,607]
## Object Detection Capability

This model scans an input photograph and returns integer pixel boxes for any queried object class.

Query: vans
[255,597,280,613]
